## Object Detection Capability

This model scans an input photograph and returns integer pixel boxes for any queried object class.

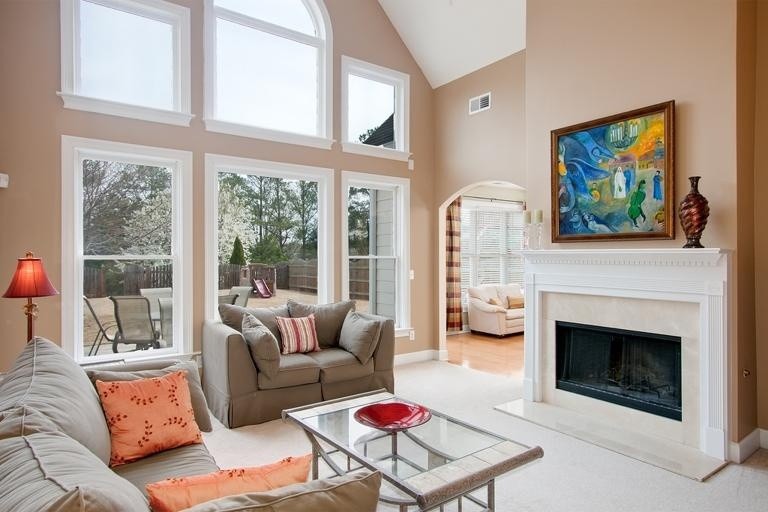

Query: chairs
[83,285,254,356]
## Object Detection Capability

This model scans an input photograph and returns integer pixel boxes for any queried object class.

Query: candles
[522,209,531,224]
[534,208,543,223]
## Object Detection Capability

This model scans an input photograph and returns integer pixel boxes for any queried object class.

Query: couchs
[200,311,395,429]
[467,284,524,339]
[0,336,383,512]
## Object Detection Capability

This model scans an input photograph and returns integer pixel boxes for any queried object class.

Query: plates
[353,402,431,433]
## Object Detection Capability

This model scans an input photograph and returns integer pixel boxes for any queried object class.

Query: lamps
[0,251,61,344]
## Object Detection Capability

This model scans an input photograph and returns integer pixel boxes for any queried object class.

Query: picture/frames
[549,99,676,242]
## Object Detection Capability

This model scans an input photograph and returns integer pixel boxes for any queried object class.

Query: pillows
[86,359,213,468]
[217,298,383,381]
[468,287,524,309]
[143,451,382,511]
